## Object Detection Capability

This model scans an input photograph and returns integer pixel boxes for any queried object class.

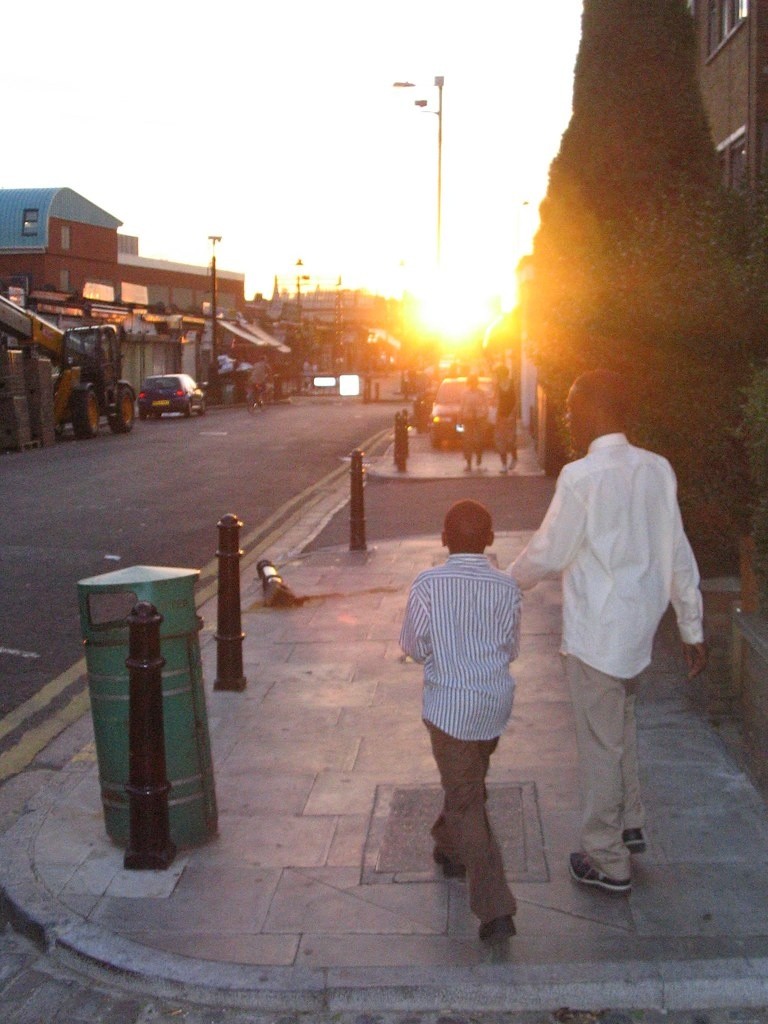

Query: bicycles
[246,374,272,413]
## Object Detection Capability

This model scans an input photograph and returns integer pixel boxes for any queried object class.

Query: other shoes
[509,458,516,469]
[622,829,644,853]
[480,914,516,941]
[500,462,507,472]
[433,844,466,879]
[463,464,471,471]
[476,465,483,478]
[568,852,632,897]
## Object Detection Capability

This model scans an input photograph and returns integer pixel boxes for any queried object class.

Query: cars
[137,374,208,422]
[427,375,499,451]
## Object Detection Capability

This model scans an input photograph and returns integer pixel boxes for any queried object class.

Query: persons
[399,500,523,942]
[500,369,708,896]
[247,355,271,404]
[457,367,518,473]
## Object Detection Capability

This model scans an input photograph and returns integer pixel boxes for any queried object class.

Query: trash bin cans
[75,563,215,852]
[222,382,237,406]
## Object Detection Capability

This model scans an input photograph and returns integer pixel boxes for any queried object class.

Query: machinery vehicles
[0,295,136,441]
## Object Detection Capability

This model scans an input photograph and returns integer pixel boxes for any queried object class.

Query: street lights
[394,72,444,257]
[206,234,223,370]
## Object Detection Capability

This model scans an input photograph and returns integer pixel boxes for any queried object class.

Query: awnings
[217,317,291,353]
